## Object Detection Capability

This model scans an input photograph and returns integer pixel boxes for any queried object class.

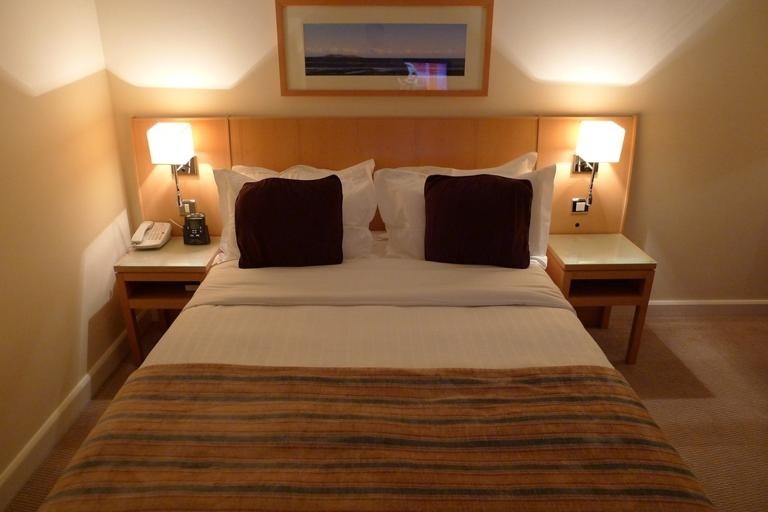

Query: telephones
[130,220,172,250]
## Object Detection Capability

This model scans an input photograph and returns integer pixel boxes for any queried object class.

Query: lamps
[572,121,626,214]
[146,122,198,214]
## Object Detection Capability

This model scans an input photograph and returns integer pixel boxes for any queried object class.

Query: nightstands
[114,235,222,367]
[546,233,658,365]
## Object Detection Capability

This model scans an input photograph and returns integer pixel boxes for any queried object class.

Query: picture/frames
[275,0,494,97]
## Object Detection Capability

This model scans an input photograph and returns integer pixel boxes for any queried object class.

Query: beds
[34,115,718,512]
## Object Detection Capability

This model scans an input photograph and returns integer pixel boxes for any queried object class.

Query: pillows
[234,174,344,268]
[214,168,377,265]
[395,151,539,175]
[424,174,534,269]
[232,159,376,182]
[373,164,556,269]
